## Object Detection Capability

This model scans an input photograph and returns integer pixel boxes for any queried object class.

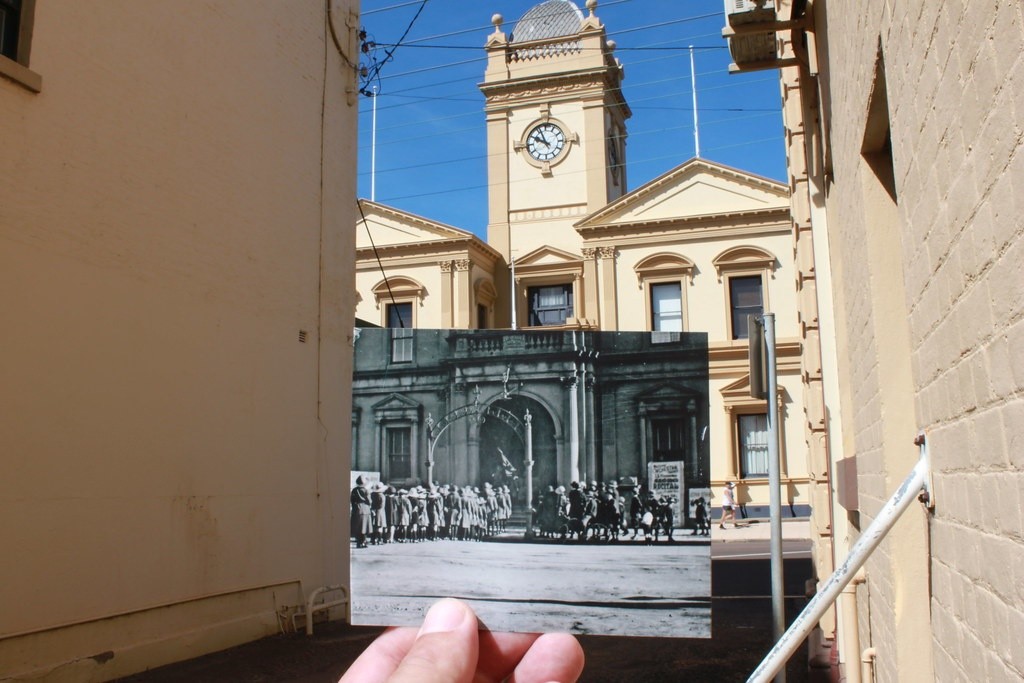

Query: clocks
[520,116,572,168]
[608,128,619,179]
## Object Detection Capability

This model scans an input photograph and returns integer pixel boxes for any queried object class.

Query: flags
[498,448,519,487]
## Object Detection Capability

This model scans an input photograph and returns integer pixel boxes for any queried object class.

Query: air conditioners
[724,0,778,65]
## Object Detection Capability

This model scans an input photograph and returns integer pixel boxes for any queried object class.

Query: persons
[532,476,710,544]
[337,596,585,683]
[720,481,743,529]
[350,474,512,548]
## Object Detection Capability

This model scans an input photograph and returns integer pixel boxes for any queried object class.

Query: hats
[448,485,458,492]
[409,488,419,497]
[473,487,480,493]
[608,480,618,487]
[483,482,492,489]
[725,482,733,485]
[570,481,581,488]
[477,497,487,504]
[371,482,387,492]
[416,486,426,492]
[356,475,369,486]
[398,489,408,494]
[555,486,565,494]
[457,488,465,497]
[545,485,554,493]
[386,486,397,494]
[503,485,510,493]
[598,482,607,488]
[589,480,598,487]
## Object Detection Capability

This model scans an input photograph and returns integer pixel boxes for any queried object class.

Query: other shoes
[540,530,711,544]
[734,523,741,527]
[720,524,726,529]
[356,529,508,548]
